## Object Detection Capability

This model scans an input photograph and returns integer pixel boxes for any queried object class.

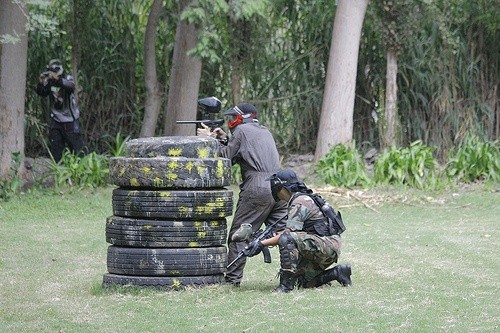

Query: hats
[266,169,298,194]
[224,102,258,120]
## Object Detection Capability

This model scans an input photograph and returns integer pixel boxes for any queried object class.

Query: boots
[321,264,352,287]
[269,270,296,295]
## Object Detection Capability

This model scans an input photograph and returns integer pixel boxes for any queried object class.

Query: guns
[226,214,289,269]
[176,96,229,145]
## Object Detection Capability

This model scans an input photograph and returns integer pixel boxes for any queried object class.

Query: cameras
[43,72,49,78]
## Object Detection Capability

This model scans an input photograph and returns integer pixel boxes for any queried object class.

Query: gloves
[244,240,265,258]
[246,230,274,244]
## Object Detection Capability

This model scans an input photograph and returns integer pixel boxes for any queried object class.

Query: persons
[36,59,85,164]
[241,169,352,292]
[197,103,288,286]
